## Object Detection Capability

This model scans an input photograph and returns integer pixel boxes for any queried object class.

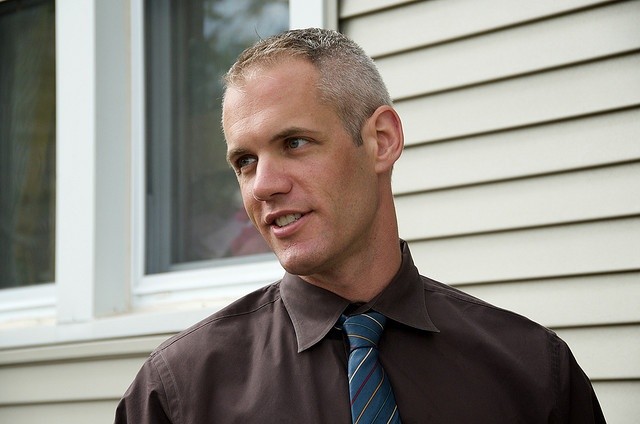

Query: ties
[341,312,402,424]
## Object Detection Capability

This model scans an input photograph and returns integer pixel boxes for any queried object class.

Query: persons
[112,28,606,424]
